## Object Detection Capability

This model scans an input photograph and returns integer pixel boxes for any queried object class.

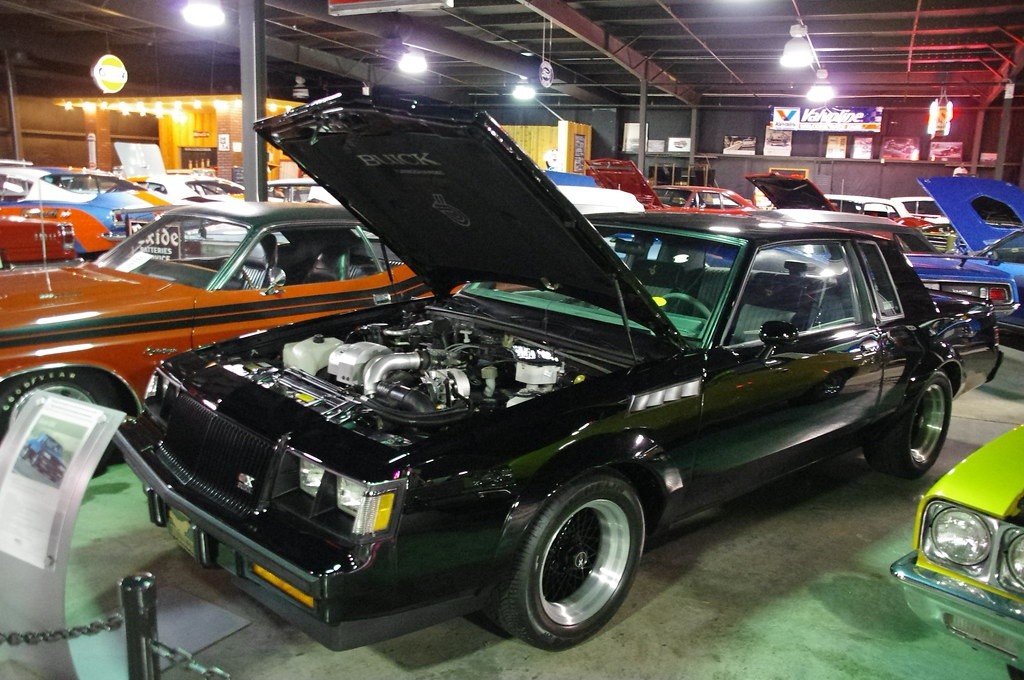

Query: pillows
[643,286,672,298]
[732,304,796,344]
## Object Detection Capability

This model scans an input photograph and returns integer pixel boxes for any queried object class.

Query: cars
[0,161,342,266]
[106,88,1022,645]
[541,148,1024,338]
[888,426,1024,678]
[0,198,544,479]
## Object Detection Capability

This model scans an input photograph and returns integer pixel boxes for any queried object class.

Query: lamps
[780,25,813,67]
[513,80,536,100]
[808,69,835,102]
